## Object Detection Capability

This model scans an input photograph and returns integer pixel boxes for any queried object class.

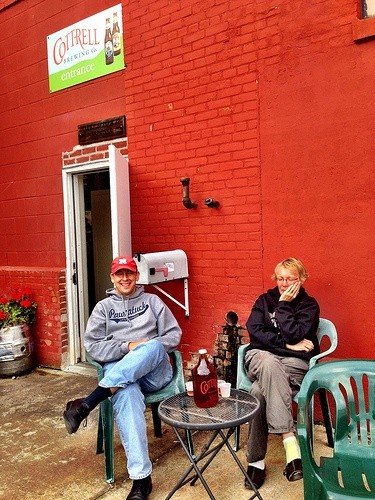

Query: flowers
[0,287,38,330]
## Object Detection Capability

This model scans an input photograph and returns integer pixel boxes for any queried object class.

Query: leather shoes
[283,459,303,481]
[126,477,152,500]
[245,464,266,488]
[63,398,90,434]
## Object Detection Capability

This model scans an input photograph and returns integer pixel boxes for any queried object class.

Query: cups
[219,383,231,398]
[217,380,225,394]
[186,381,194,396]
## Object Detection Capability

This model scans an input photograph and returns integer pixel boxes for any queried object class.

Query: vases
[0,324,35,376]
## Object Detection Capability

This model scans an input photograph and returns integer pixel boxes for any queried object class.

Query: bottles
[192,349,218,407]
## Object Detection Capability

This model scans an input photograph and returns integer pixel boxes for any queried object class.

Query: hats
[111,256,137,274]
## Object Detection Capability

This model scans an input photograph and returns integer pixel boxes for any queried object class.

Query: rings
[290,290,293,293]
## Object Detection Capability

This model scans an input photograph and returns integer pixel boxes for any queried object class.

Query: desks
[158,389,264,500]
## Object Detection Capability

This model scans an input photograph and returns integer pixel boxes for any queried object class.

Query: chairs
[85,349,194,484]
[297,358,375,500]
[234,318,338,451]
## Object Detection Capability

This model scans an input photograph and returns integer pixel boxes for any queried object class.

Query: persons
[243,257,321,489]
[62,256,183,500]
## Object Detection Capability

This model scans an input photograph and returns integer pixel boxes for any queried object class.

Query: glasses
[277,277,299,283]
